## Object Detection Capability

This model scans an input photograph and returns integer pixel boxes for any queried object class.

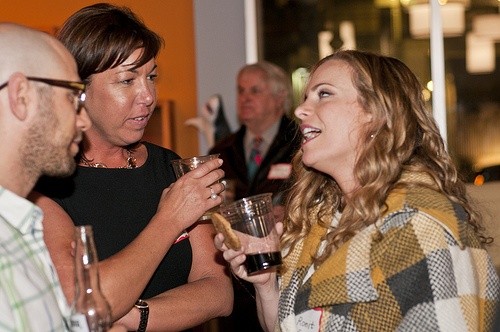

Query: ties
[248,136,262,182]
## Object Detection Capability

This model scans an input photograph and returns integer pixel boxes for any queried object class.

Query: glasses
[0,77,87,115]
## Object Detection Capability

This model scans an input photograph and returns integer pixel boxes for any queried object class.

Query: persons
[205,200,207,201]
[27,2,235,332]
[0,23,128,332]
[212,50,500,332]
[205,60,303,332]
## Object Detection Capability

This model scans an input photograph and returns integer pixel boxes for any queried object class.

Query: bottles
[70,226,114,332]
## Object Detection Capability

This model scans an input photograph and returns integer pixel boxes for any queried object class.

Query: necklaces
[76,144,139,170]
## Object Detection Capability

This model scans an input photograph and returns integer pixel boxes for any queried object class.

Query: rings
[206,186,218,201]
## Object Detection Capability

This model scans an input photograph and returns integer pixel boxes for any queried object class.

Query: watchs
[134,299,150,332]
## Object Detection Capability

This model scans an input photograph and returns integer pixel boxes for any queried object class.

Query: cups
[216,192,283,277]
[170,154,221,222]
